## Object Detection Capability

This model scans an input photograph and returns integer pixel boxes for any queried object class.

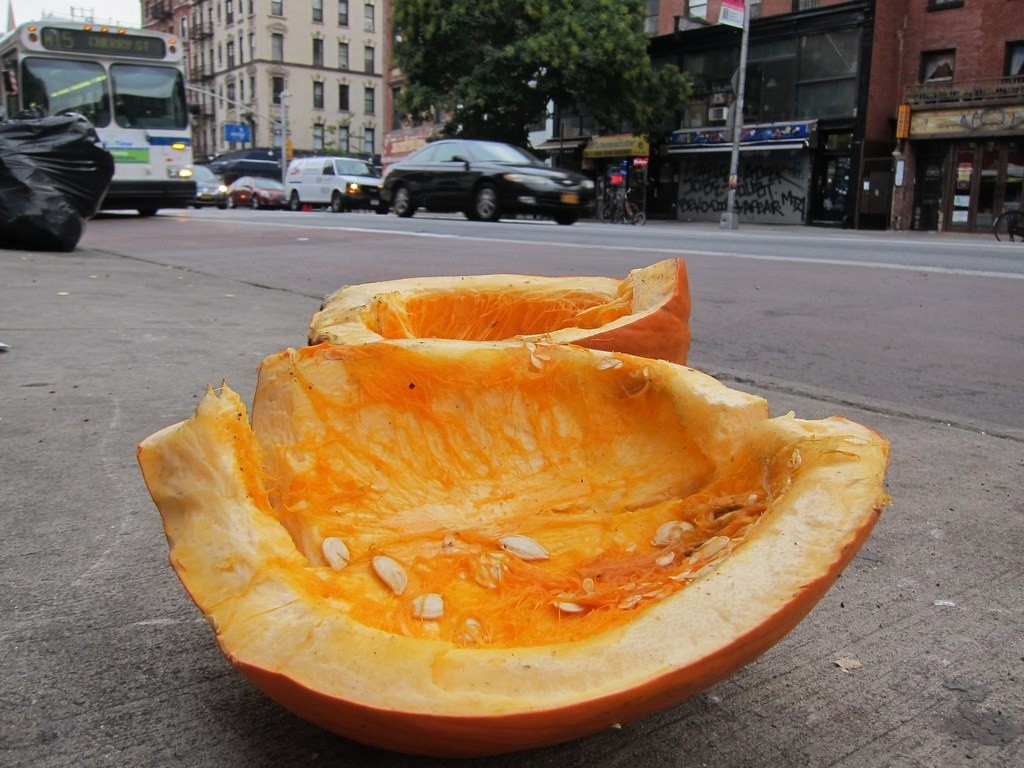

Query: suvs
[205,146,295,183]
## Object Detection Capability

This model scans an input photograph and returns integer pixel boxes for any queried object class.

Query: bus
[0,18,201,218]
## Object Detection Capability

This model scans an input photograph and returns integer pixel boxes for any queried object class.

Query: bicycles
[600,186,647,228]
[992,206,1024,242]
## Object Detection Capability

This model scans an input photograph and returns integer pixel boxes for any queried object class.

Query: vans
[284,155,384,213]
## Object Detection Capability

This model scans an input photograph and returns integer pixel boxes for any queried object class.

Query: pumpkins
[136,335,891,755]
[307,257,691,369]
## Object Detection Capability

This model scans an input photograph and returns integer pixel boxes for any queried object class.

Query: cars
[379,138,596,225]
[192,164,229,209]
[226,175,286,210]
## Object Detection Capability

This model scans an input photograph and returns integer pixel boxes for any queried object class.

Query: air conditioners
[708,106,727,121]
